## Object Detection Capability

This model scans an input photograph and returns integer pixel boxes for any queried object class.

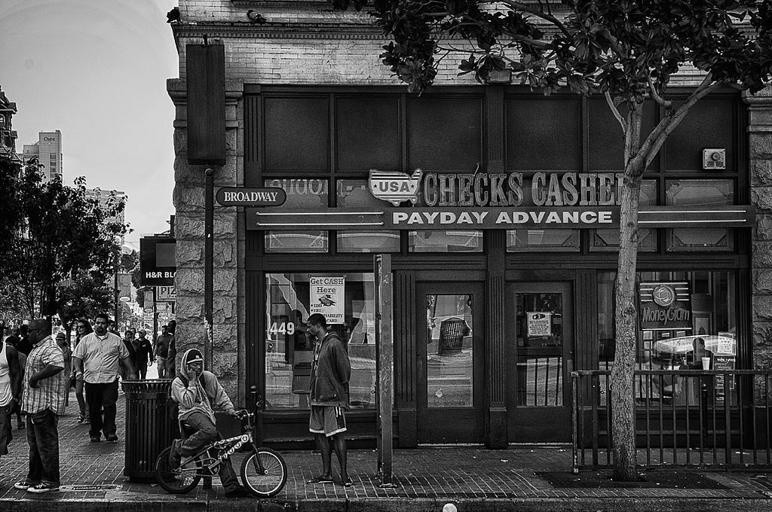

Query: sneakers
[169,440,182,468]
[77,411,85,423]
[27,480,59,493]
[85,417,90,424]
[90,435,100,442]
[225,485,248,498]
[14,479,40,489]
[106,433,118,442]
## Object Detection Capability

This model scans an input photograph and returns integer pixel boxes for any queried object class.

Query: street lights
[109,234,122,336]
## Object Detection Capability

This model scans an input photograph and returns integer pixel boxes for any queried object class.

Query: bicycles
[154,384,287,497]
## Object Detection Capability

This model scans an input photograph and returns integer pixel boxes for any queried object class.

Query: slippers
[307,474,334,484]
[341,472,354,487]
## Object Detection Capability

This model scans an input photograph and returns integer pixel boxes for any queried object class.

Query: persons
[169,348,250,498]
[289,309,316,407]
[686,337,716,405]
[345,317,373,406]
[306,313,354,486]
[0,313,176,494]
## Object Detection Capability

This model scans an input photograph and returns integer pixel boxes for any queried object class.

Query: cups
[701,357,711,370]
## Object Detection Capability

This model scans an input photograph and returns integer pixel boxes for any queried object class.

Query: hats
[187,349,203,363]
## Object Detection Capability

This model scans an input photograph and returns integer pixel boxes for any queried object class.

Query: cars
[124,303,170,348]
[649,326,735,401]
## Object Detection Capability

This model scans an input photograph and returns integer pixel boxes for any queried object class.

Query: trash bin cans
[122,379,177,482]
